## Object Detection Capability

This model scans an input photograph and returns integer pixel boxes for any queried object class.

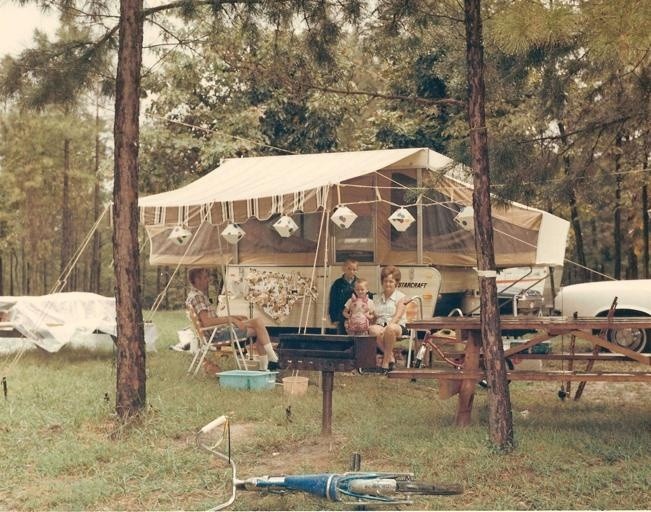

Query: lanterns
[388,209,415,231]
[170,226,192,246]
[455,207,474,230]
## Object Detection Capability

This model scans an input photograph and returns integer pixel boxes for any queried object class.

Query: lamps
[387,207,417,233]
[168,225,193,246]
[453,206,476,232]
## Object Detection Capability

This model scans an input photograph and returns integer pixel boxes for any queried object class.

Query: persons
[368,264,408,370]
[185,267,279,371]
[328,258,373,335]
[342,278,374,335]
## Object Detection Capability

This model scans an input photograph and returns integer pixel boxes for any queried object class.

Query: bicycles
[397,294,517,390]
[192,414,465,512]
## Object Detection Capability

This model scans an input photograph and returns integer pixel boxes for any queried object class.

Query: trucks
[216,262,544,336]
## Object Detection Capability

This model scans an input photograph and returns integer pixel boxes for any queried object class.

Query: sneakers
[268,361,283,370]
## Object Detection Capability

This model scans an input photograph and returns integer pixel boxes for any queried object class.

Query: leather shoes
[381,358,397,376]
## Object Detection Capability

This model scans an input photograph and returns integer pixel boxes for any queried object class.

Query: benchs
[388,348,651,428]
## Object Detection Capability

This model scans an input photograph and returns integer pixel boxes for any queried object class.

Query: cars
[552,276,651,357]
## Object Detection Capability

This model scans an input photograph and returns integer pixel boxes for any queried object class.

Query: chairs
[182,303,250,382]
[398,329,419,369]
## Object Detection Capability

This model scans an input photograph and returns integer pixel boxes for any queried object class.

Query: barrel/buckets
[282,376,310,395]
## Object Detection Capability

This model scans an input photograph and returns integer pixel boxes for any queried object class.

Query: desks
[407,314,651,427]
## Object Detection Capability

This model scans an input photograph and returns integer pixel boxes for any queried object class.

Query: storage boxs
[216,368,281,393]
